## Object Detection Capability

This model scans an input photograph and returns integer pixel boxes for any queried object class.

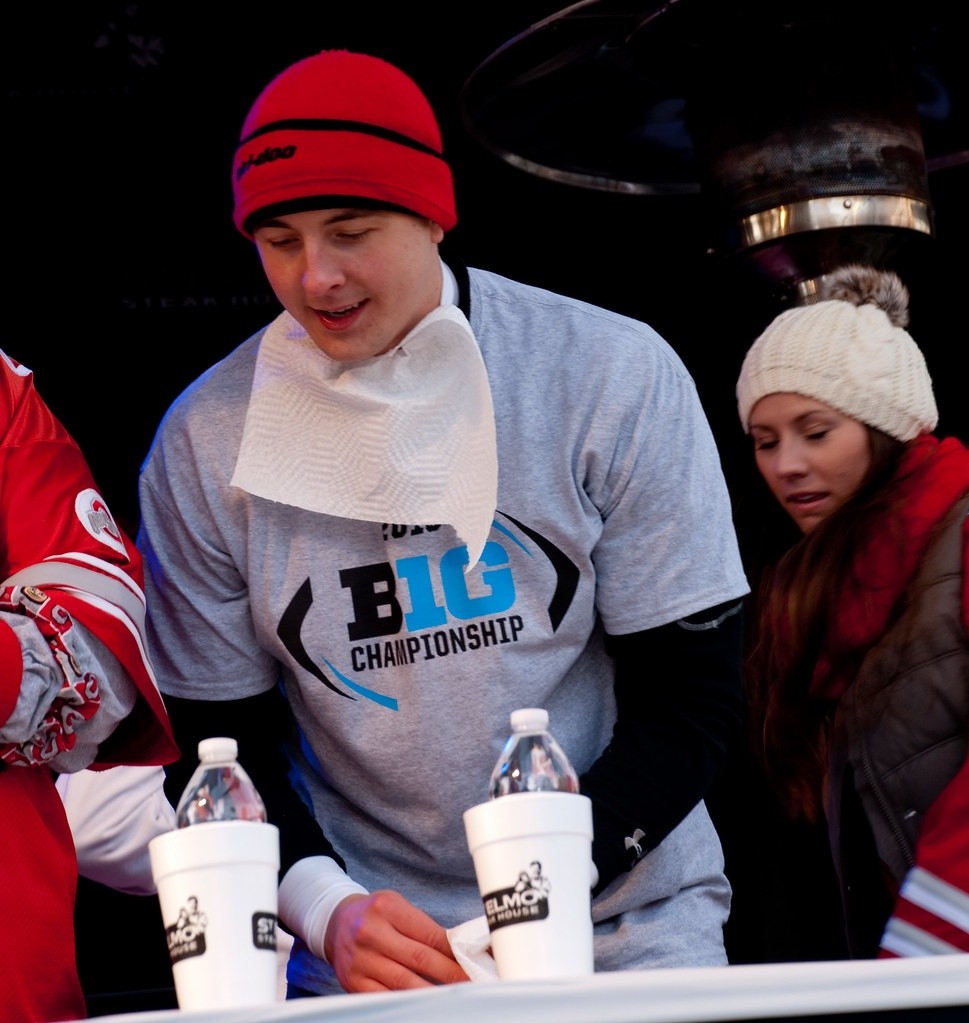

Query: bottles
[488,707,581,801]
[174,736,270,827]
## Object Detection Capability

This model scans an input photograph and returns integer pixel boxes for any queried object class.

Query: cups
[147,819,280,1010]
[461,790,596,979]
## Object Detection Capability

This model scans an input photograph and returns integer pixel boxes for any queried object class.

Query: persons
[136,46,753,1003]
[735,261,969,1023]
[0,338,181,1023]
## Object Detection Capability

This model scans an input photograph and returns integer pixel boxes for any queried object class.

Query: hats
[736,265,938,449]
[231,50,456,245]
